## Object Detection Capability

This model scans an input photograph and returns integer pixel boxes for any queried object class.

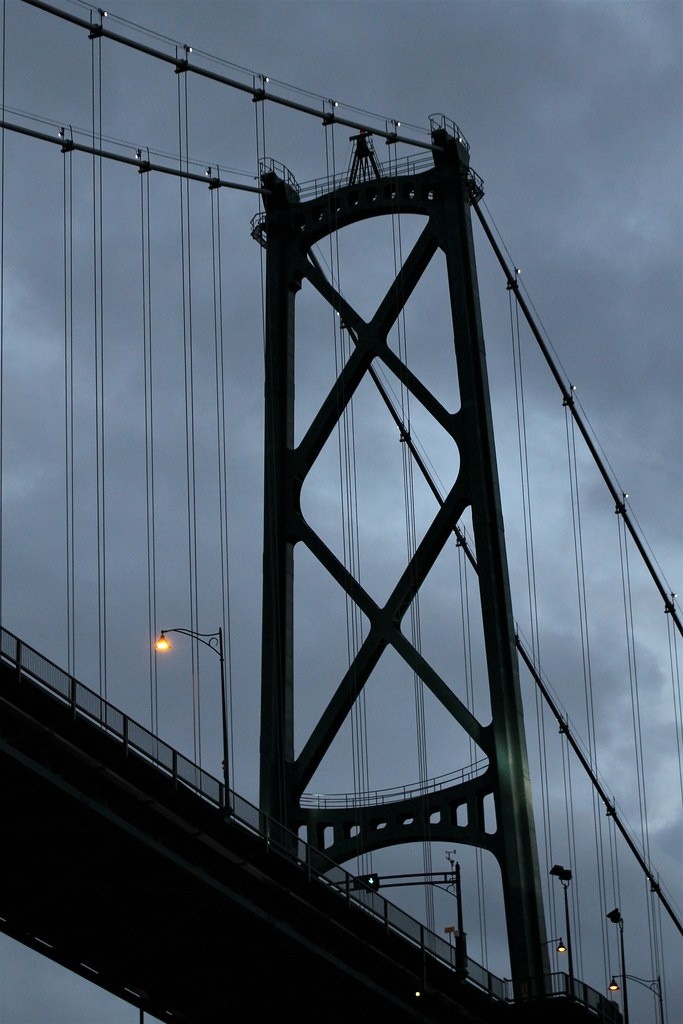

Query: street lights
[550,863,576,997]
[605,908,628,1024]
[153,627,230,816]
[605,974,664,1024]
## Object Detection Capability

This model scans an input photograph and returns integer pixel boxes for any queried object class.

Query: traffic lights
[353,873,378,891]
